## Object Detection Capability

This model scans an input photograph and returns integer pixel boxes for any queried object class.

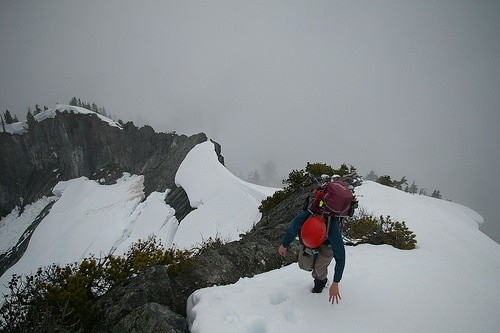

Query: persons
[277,206,347,306]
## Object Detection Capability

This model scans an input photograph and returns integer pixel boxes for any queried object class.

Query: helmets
[301,217,327,249]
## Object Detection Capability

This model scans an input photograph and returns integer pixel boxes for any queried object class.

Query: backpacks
[310,178,359,219]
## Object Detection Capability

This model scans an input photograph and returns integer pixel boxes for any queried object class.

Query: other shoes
[311,278,327,293]
